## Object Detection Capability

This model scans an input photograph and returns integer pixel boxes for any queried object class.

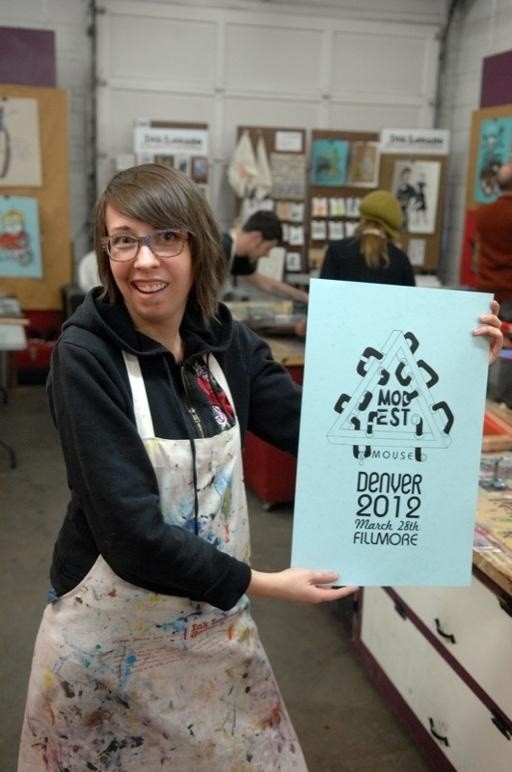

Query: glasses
[99,228,188,264]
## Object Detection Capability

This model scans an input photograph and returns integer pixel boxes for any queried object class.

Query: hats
[357,189,406,243]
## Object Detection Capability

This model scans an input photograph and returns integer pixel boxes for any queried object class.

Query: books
[276,191,364,326]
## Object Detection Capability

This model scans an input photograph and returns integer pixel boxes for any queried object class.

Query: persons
[467,165,512,406]
[214,209,314,303]
[12,162,504,772]
[295,188,414,339]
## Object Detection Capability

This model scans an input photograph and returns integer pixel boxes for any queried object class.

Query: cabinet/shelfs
[321,546,512,770]
